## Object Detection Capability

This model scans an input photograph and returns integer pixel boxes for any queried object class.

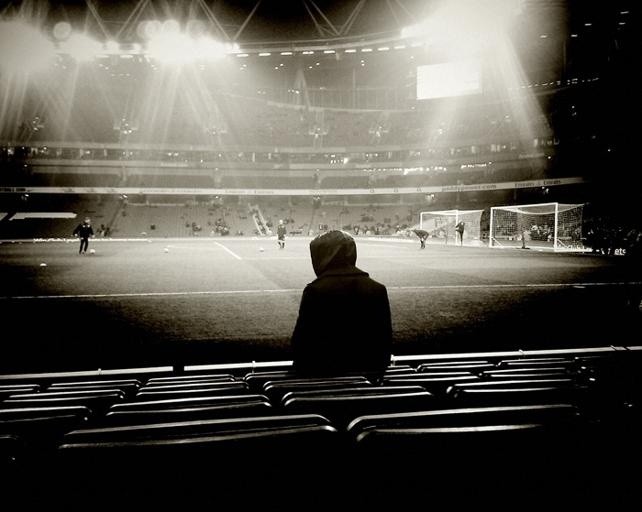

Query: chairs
[0,346,642,512]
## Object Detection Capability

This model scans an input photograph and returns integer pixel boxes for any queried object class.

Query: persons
[95,195,491,239]
[491,195,642,258]
[455,220,466,247]
[410,228,429,248]
[72,216,95,255]
[276,219,286,248]
[281,226,393,381]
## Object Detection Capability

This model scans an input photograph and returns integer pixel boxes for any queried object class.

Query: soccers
[164,248,168,252]
[90,249,95,254]
[260,249,264,253]
